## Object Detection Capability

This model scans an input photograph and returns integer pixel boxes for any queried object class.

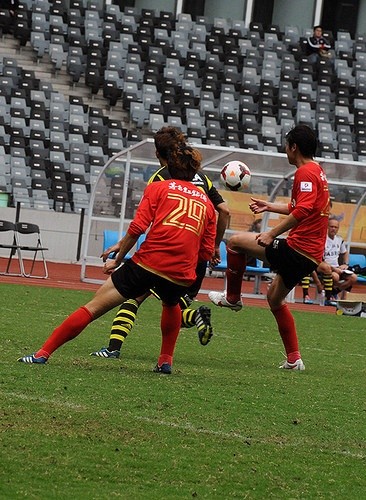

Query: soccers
[220,161,251,191]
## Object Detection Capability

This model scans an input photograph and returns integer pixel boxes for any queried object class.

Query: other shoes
[303,295,313,303]
[319,290,325,299]
[326,291,339,303]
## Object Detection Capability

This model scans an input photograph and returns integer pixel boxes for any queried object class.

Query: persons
[305,24,333,55]
[17,145,216,374]
[249,201,358,306]
[209,126,330,371]
[91,126,231,357]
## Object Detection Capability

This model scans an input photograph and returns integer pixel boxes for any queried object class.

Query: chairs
[346,254,366,281]
[206,241,228,270]
[0,0,366,221]
[243,259,270,294]
[136,234,146,251]
[103,230,132,271]
[8,222,49,279]
[0,220,24,277]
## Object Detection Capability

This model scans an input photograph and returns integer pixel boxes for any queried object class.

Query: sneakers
[208,290,242,313]
[153,362,172,374]
[17,355,48,365]
[89,348,120,358]
[278,358,306,372]
[195,304,214,346]
